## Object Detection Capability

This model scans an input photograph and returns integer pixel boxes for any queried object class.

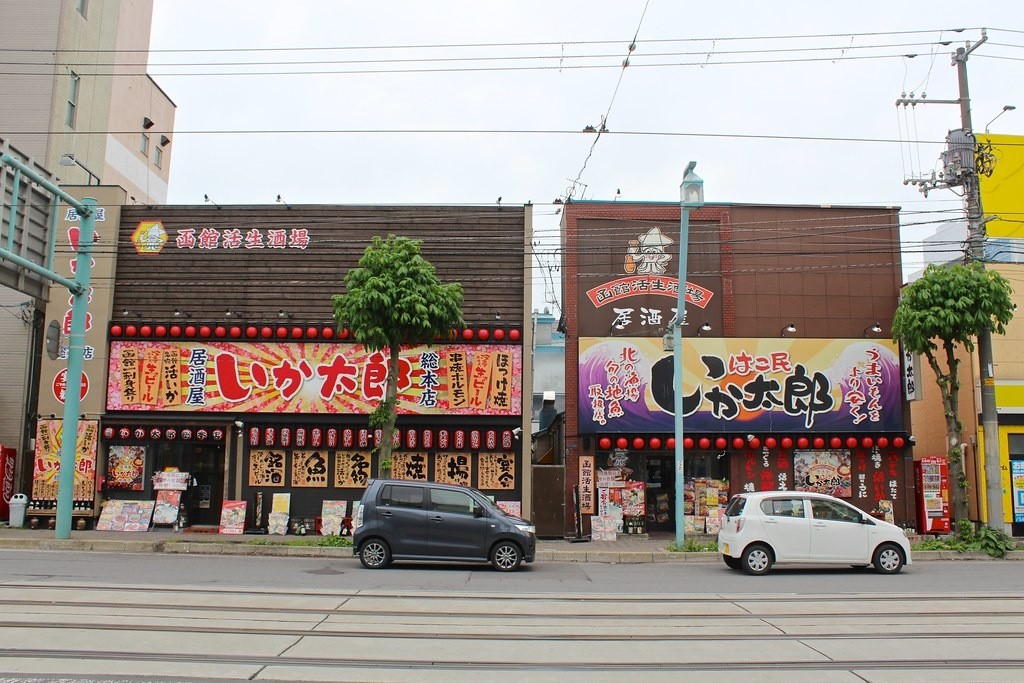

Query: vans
[718,492,911,575]
[353,479,536,571]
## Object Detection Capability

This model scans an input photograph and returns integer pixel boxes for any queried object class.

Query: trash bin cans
[7,493,28,528]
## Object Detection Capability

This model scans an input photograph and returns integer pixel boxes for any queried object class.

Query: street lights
[664,162,704,550]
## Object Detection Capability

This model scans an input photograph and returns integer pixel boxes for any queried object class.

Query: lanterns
[104,428,115,439]
[135,428,147,440]
[249,427,260,446]
[119,428,131,439]
[264,428,276,447]
[281,428,292,447]
[296,428,306,447]
[438,430,448,449]
[327,429,337,448]
[151,429,161,440]
[342,429,353,448]
[392,428,401,448]
[196,430,208,440]
[358,429,368,448]
[501,430,512,450]
[486,430,496,449]
[422,430,433,449]
[181,429,192,440]
[454,430,465,448]
[470,430,480,449]
[212,429,224,441]
[312,428,322,447]
[407,430,417,449]
[165,429,177,440]
[374,429,382,447]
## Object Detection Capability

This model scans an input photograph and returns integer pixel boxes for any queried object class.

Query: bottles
[175,520,179,533]
[637,517,643,534]
[342,523,349,536]
[29,517,38,530]
[922,465,940,490]
[295,519,307,535]
[627,518,633,534]
[75,518,87,531]
[74,501,92,511]
[47,516,56,530]
[27,500,57,510]
[895,518,916,533]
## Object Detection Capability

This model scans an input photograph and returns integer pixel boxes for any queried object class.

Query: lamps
[495,311,501,319]
[224,306,242,319]
[864,322,882,338]
[696,320,712,338]
[512,427,523,435]
[278,309,294,318]
[909,436,917,441]
[609,319,625,337]
[780,321,796,337]
[747,434,755,442]
[276,194,291,210]
[716,450,726,459]
[235,421,245,429]
[204,194,221,210]
[130,196,152,209]
[122,308,141,317]
[175,308,193,317]
[332,310,336,317]
